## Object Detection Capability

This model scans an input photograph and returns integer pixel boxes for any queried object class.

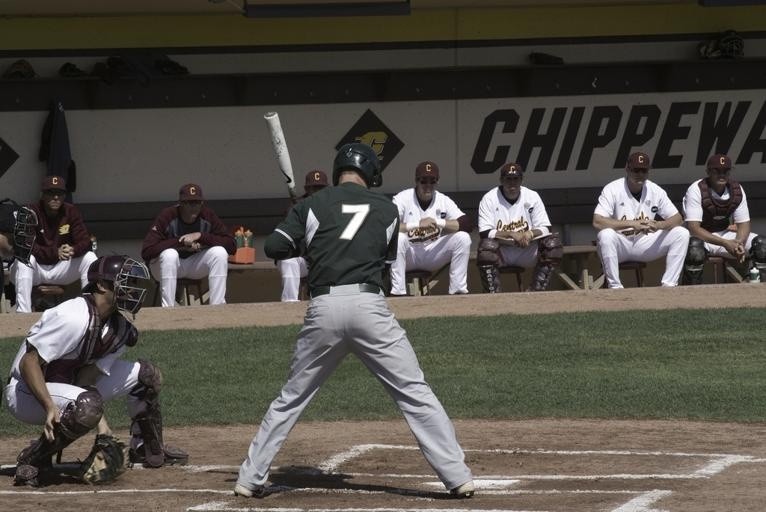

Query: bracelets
[530,230,534,239]
[406,221,420,230]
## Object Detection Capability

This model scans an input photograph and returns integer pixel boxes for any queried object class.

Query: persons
[390,161,472,296]
[10,173,99,314]
[1,197,40,267]
[143,182,237,308]
[274,168,330,300]
[477,162,563,293]
[592,151,690,290]
[682,154,766,282]
[6,256,188,488]
[234,142,475,499]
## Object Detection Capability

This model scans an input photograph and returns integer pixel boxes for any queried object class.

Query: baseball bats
[265,112,298,205]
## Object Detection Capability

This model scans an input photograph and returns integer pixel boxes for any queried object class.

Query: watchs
[435,218,446,228]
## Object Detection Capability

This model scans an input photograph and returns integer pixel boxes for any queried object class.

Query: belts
[307,282,388,299]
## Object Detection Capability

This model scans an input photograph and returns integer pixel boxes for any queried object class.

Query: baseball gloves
[77,434,131,485]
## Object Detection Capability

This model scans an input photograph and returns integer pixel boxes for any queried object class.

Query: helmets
[0,200,38,265]
[86,254,150,315]
[332,143,384,189]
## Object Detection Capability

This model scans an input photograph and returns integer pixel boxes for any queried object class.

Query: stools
[404,270,433,296]
[495,264,526,291]
[33,285,66,313]
[298,274,312,300]
[600,260,648,288]
[149,278,205,306]
[704,256,725,285]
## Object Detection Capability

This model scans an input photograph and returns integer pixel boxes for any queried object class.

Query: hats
[707,153,732,172]
[304,170,328,187]
[179,183,205,202]
[416,161,439,181]
[39,175,67,192]
[500,162,523,178]
[627,152,651,169]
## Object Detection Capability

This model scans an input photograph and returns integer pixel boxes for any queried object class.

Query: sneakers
[128,441,189,466]
[13,455,55,488]
[450,480,475,500]
[233,479,267,499]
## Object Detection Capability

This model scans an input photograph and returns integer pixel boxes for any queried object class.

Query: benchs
[177,245,606,306]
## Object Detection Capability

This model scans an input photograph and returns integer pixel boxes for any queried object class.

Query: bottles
[234,230,243,248]
[88,233,98,255]
[243,229,253,248]
[749,267,761,284]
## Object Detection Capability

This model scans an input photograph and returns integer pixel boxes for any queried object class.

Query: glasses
[633,168,647,174]
[421,180,436,185]
[45,190,66,196]
